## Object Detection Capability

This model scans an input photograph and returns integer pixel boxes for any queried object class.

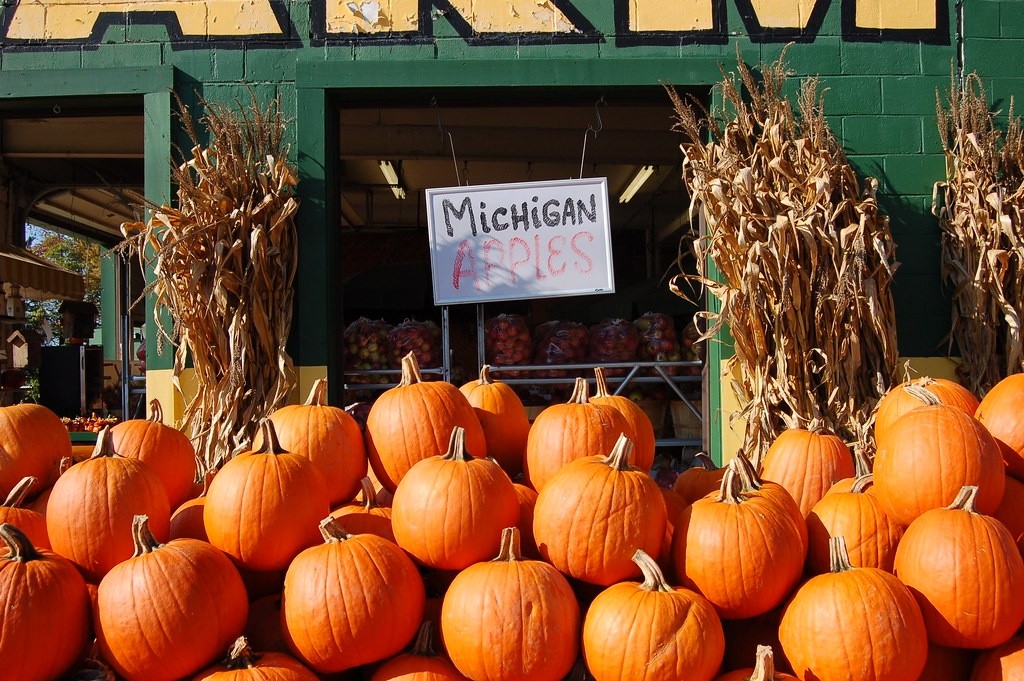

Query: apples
[340,313,709,420]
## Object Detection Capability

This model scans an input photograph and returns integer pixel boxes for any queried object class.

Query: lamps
[379,161,407,200]
[615,164,658,205]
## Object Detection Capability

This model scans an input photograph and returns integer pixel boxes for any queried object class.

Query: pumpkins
[0,352,1024,681]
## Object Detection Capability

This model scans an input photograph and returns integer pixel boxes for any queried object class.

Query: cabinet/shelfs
[484,359,710,493]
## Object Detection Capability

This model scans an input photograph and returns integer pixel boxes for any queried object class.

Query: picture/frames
[425,177,615,305]
